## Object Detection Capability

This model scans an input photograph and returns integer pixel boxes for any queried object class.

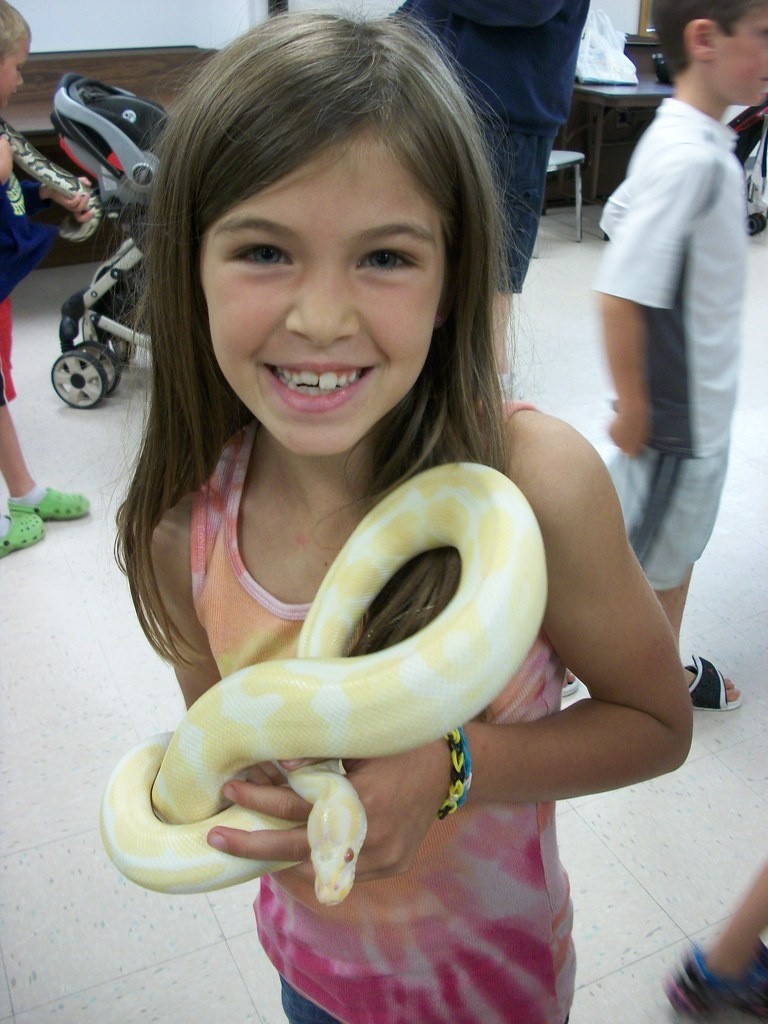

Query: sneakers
[663,936,768,1024]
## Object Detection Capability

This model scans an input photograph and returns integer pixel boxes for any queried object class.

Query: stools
[543,149,587,242]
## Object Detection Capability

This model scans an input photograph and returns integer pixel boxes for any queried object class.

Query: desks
[559,71,673,202]
[0,97,63,148]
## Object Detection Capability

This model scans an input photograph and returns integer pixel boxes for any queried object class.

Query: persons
[0,0,92,559]
[398,0,591,374]
[561,0,768,710]
[664,863,768,1024]
[112,9,692,1024]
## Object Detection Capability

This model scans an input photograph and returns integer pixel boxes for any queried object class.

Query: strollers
[727,101,768,234]
[49,72,170,409]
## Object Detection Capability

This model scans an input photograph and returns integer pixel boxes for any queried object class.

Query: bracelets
[438,726,472,819]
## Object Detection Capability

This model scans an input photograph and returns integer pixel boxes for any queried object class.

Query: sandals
[0,515,46,558]
[685,656,743,711]
[561,673,579,697]
[8,487,89,520]
